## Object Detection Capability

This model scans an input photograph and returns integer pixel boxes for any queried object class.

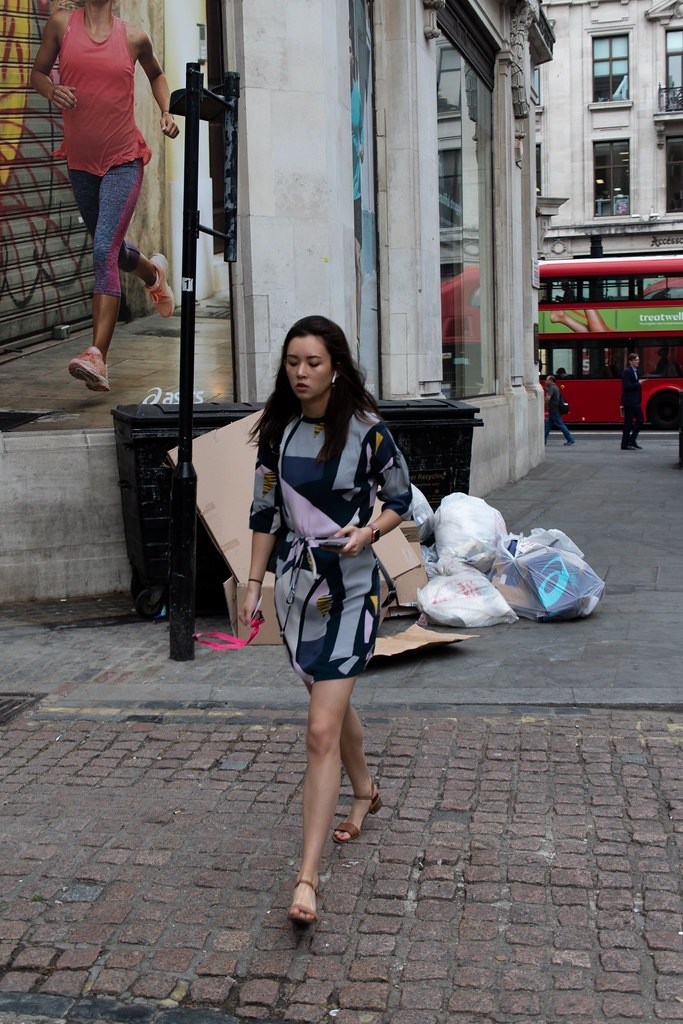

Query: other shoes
[564,441,574,445]
[629,442,641,448]
[622,445,636,450]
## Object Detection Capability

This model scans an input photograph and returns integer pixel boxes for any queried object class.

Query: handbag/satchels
[558,394,569,414]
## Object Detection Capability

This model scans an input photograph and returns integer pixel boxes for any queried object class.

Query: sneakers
[144,253,174,318]
[68,346,110,391]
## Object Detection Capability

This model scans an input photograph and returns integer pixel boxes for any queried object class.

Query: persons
[651,348,670,374]
[28,0,180,391]
[621,353,644,450]
[550,308,617,333]
[543,376,576,446]
[555,281,575,303]
[238,316,415,925]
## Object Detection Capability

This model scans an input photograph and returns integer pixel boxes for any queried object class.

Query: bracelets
[248,578,262,586]
[162,110,172,114]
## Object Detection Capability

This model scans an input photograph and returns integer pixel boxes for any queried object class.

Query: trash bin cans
[112,400,484,619]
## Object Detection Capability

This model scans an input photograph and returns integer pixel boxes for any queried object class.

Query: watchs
[367,523,381,544]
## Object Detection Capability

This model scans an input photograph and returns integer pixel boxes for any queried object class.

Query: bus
[439,259,482,386]
[536,254,683,432]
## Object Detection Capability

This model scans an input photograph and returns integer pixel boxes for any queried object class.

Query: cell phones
[319,536,350,548]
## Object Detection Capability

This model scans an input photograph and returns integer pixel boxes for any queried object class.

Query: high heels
[286,880,323,925]
[332,781,383,843]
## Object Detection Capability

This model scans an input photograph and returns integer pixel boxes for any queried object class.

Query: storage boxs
[221,521,427,646]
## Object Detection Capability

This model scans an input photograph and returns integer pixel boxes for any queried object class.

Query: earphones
[332,371,338,383]
